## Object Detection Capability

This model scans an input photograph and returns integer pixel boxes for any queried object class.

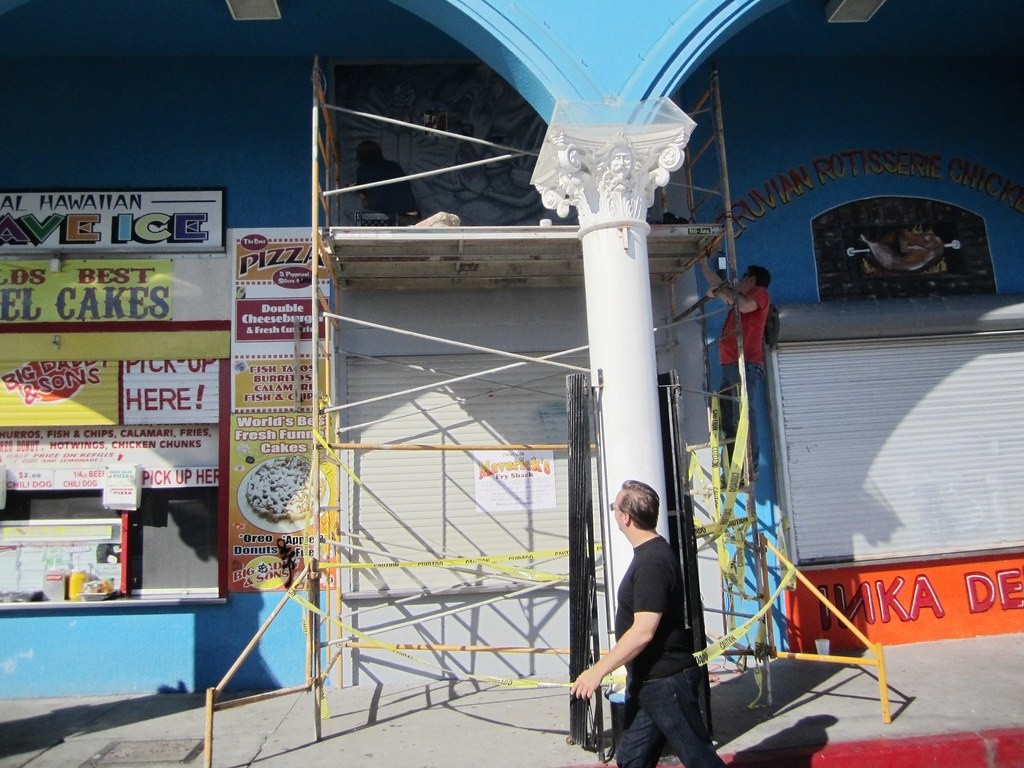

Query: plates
[237,457,329,534]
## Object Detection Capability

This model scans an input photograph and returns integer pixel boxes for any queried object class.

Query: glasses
[611,503,632,516]
[742,272,755,278]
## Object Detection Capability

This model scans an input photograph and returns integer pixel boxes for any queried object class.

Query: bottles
[68,569,89,600]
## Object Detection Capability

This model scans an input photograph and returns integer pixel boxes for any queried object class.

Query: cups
[540,219,552,227]
[815,639,830,655]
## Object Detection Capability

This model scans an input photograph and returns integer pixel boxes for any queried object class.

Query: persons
[570,480,732,768]
[340,140,420,227]
[699,252,772,487]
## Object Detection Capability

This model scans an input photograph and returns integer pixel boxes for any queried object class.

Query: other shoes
[719,469,760,489]
